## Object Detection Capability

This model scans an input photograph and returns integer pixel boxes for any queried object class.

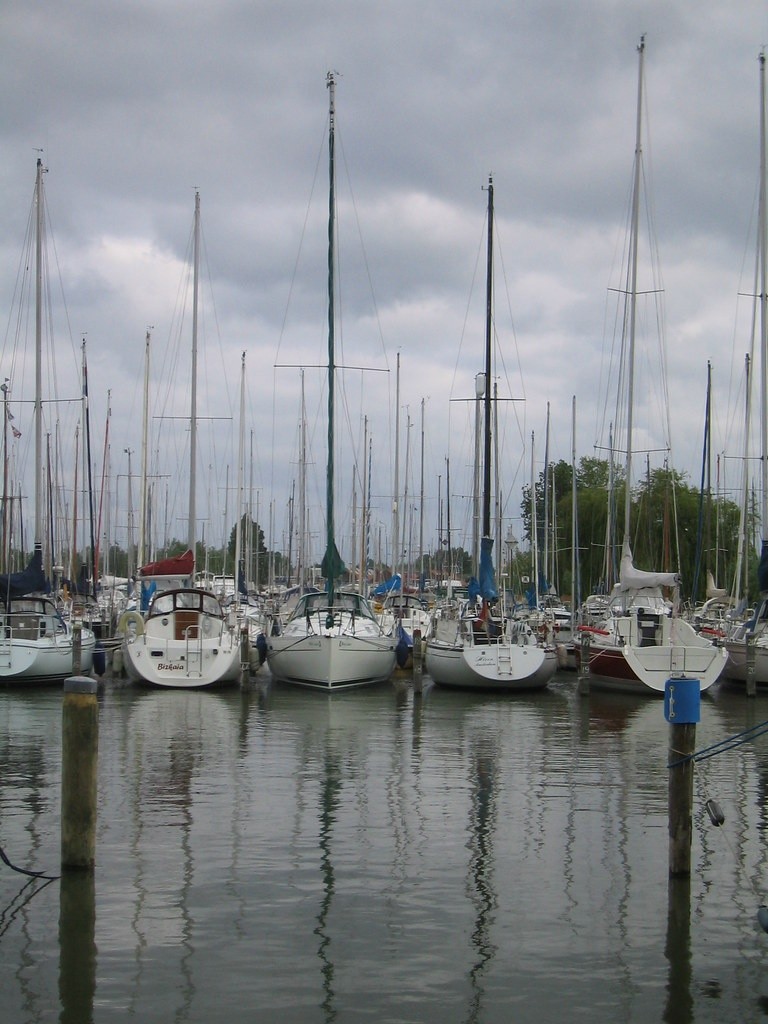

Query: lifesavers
[119,611,144,635]
[539,625,556,643]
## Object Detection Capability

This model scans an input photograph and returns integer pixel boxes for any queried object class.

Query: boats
[248,69,410,692]
[122,187,246,687]
[570,31,768,693]
[424,173,558,692]
[1,324,578,671]
[1,149,96,681]
